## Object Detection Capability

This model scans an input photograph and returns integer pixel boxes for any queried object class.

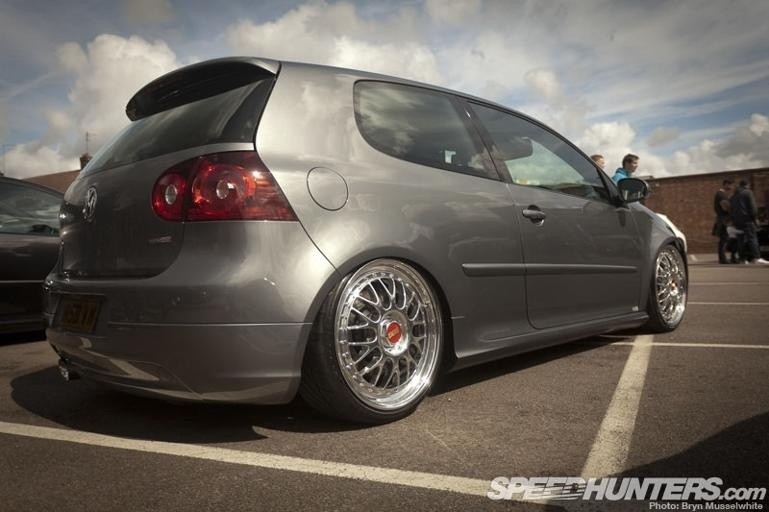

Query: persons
[714,179,735,264]
[612,154,639,183]
[730,178,769,264]
[590,154,604,168]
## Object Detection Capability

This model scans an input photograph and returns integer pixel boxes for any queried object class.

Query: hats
[41,55,690,425]
[1,173,66,339]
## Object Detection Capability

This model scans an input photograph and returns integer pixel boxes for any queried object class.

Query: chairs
[720,258,769,265]
